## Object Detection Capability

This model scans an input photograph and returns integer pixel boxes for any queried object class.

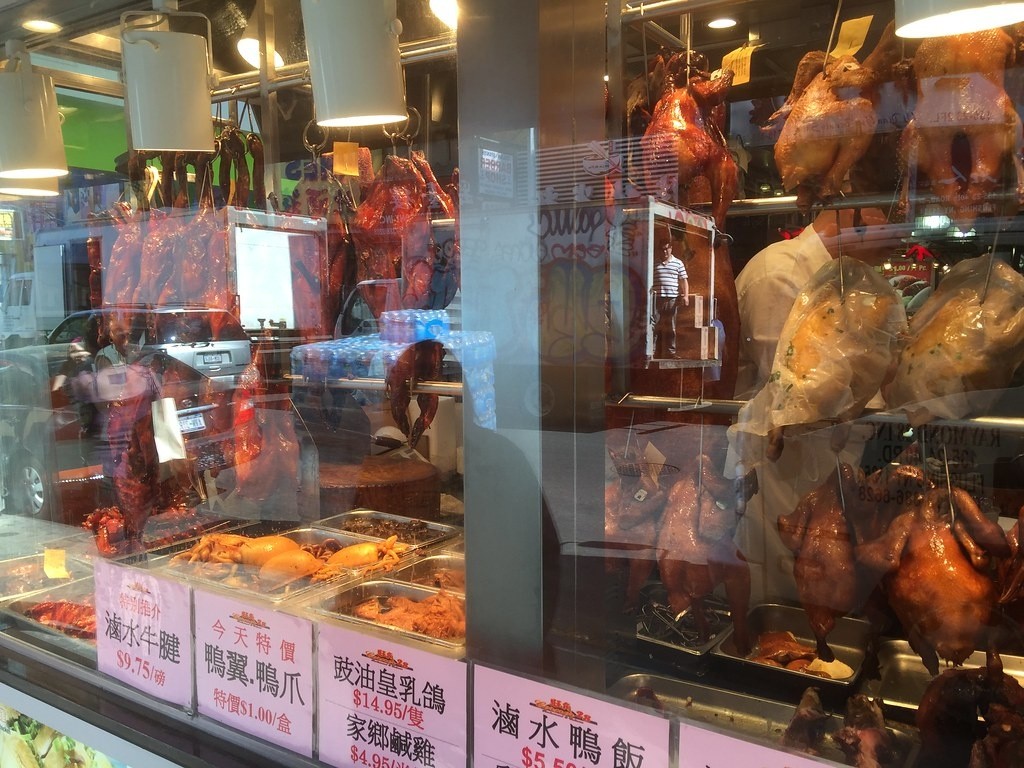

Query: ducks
[83,19,460,558]
[601,19,1024,680]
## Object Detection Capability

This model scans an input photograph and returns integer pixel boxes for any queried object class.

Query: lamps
[894,1,1024,39]
[297,0,409,128]
[237,1,299,69]
[120,0,218,153]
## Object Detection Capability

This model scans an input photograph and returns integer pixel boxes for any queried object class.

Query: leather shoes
[666,354,681,359]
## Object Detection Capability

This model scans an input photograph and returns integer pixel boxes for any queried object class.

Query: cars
[0,344,261,526]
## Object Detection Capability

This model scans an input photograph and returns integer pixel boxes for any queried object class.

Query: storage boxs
[439,534,466,558]
[299,579,468,649]
[161,525,411,604]
[1,575,98,665]
[711,598,873,712]
[383,556,467,599]
[604,674,924,768]
[624,578,735,679]
[856,639,1023,727]
[308,507,465,548]
[0,553,96,628]
[44,509,264,563]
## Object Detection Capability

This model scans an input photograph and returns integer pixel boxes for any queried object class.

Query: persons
[708,162,932,610]
[647,239,690,362]
[51,336,99,441]
[93,315,142,509]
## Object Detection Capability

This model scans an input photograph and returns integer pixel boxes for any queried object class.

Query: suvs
[0,303,251,437]
